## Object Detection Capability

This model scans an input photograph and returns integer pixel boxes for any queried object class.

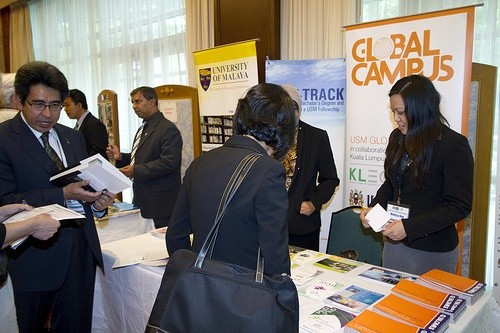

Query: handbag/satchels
[143,248,300,333]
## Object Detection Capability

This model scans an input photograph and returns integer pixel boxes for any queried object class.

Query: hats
[278,84,303,119]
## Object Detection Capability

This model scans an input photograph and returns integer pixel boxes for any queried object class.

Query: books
[338,268,487,333]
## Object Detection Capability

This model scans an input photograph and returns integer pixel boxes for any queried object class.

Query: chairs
[325,205,383,265]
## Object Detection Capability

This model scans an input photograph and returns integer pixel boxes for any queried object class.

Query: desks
[91,225,497,333]
[94,200,143,244]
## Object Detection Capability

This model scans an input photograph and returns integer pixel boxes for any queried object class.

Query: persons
[106,86,183,228]
[360,74,473,276]
[144,83,300,333]
[279,84,340,252]
[0,62,117,333]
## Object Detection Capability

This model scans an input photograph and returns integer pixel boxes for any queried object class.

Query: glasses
[25,98,64,112]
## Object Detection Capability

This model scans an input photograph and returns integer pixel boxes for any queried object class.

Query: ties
[73,123,79,131]
[129,121,147,181]
[40,130,66,173]
